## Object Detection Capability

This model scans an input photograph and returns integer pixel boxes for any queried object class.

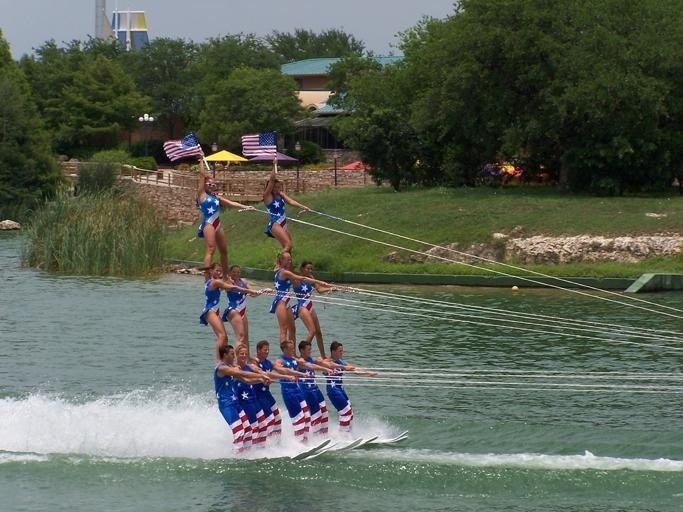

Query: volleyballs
[512,286,519,292]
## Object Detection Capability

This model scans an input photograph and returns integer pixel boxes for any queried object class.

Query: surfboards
[292,431,409,459]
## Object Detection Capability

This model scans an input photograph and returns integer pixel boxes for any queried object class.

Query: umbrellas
[195,148,250,171]
[248,152,302,171]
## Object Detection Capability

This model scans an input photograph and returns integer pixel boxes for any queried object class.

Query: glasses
[205,183,216,187]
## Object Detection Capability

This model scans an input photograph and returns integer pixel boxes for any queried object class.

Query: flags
[240,131,278,158]
[163,133,204,162]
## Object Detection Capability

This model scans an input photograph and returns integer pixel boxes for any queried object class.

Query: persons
[200,262,257,360]
[294,261,338,360]
[196,157,256,271]
[214,342,377,453]
[221,266,273,351]
[262,158,310,253]
[270,251,329,352]
